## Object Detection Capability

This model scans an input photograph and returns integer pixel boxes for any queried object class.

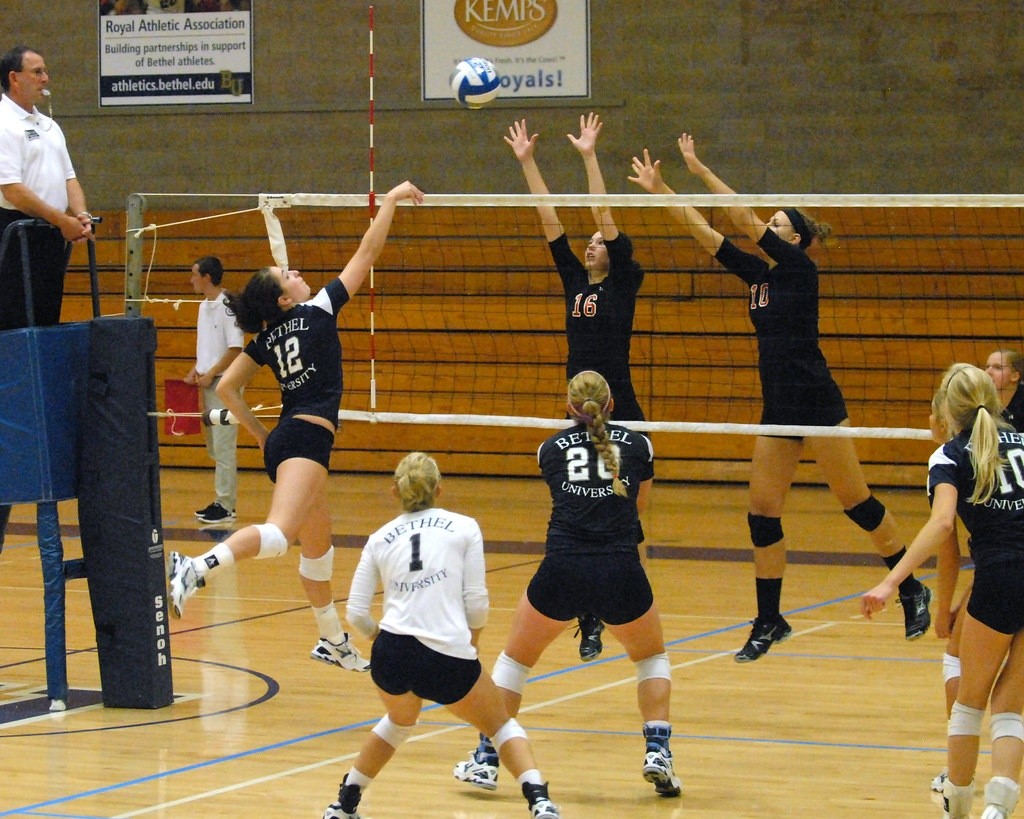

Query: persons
[0,45,96,331]
[861,347,1024,819]
[183,255,245,524]
[168,180,425,672]
[628,133,933,662]
[504,113,654,662]
[320,452,561,819]
[453,370,682,795]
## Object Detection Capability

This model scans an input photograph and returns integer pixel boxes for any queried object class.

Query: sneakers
[167,550,205,620]
[642,721,681,794]
[310,632,371,673]
[522,781,561,819]
[322,773,361,819]
[895,580,933,641]
[734,614,792,663]
[567,613,606,663]
[453,747,498,791]
[194,501,236,518]
[198,504,232,523]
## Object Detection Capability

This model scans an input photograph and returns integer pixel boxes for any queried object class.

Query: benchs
[48,211,1024,487]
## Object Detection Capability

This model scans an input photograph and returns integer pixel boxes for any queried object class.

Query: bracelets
[82,212,92,219]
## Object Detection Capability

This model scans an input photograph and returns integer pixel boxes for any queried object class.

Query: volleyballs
[449,57,502,111]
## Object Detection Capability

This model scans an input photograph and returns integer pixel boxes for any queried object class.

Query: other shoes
[940,795,969,819]
[932,771,977,800]
[981,805,1006,819]
